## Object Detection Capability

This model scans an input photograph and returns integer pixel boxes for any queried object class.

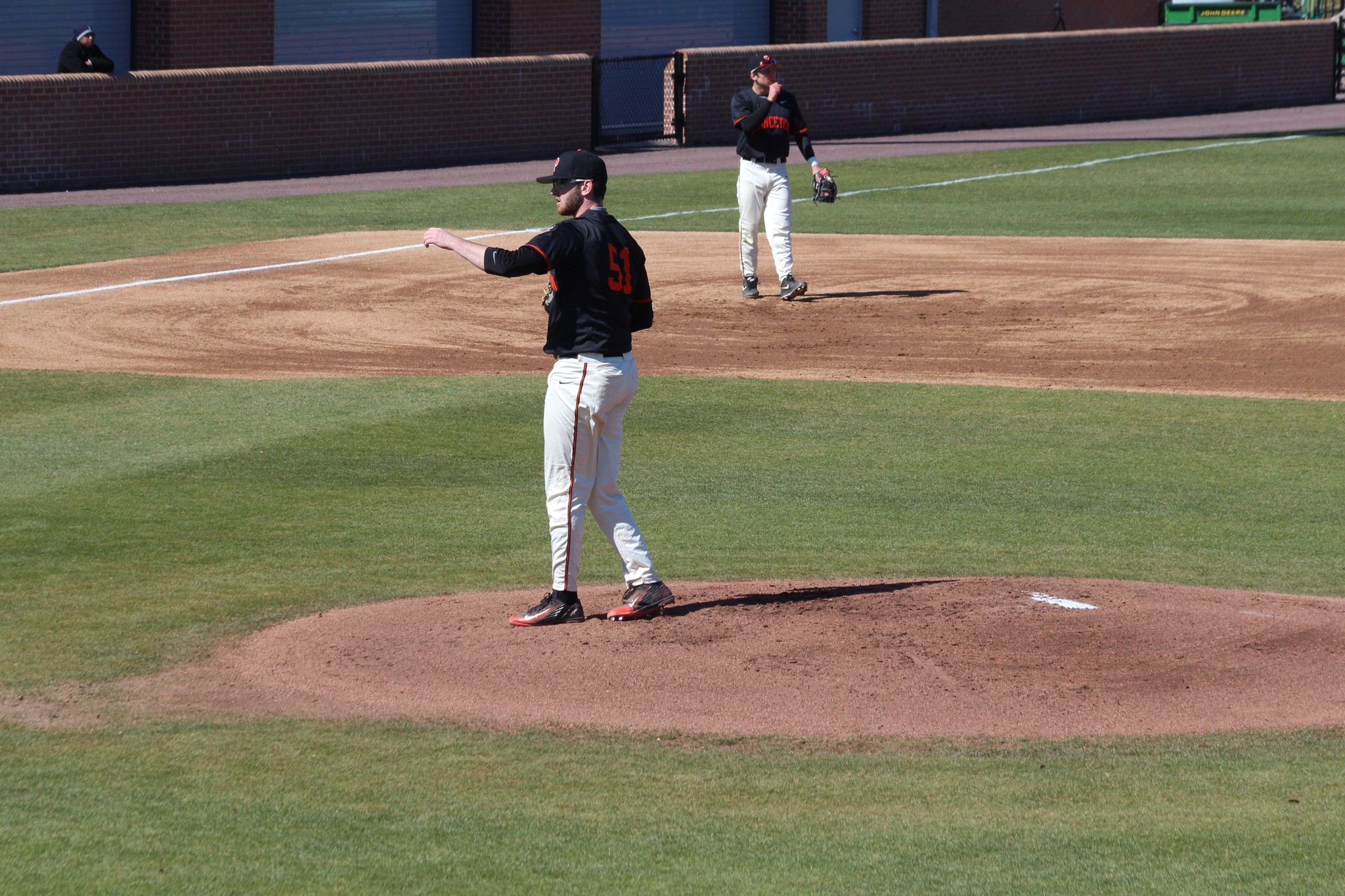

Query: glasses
[751,58,775,74]
[554,178,594,189]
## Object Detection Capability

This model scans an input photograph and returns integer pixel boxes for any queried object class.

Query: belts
[743,156,786,164]
[553,352,623,359]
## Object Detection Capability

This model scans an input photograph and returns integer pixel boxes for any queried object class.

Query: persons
[57,24,115,73]
[420,145,677,629]
[730,52,835,300]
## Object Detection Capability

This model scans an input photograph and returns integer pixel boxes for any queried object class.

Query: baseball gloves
[812,168,837,203]
[541,278,554,314]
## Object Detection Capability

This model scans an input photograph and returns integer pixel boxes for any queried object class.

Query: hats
[750,54,777,73]
[539,150,607,183]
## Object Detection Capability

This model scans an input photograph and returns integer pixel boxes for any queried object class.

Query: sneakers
[780,275,807,301]
[607,580,675,621]
[508,590,584,627]
[742,276,759,298]
